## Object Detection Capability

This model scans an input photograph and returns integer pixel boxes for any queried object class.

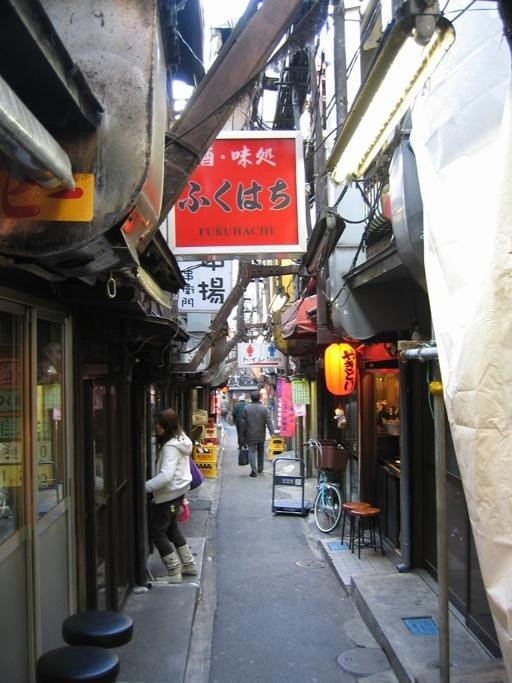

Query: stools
[351,508,384,558]
[36,645,120,683]
[341,502,372,550]
[63,611,133,648]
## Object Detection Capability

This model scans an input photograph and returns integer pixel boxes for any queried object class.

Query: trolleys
[271,456,312,516]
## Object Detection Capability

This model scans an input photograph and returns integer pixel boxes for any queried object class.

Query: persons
[145,407,199,584]
[221,390,275,477]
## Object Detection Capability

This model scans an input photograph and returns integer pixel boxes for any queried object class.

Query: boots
[156,553,182,583]
[176,544,198,576]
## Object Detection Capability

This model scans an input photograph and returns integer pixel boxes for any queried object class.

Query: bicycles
[303,439,351,533]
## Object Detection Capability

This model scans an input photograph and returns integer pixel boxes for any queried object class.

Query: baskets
[318,446,348,471]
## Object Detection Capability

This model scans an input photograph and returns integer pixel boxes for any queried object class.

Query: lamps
[321,0,456,183]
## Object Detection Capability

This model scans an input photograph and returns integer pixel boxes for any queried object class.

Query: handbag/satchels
[188,457,204,491]
[239,449,249,465]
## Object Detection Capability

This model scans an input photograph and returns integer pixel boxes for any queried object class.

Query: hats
[251,391,260,398]
[238,396,245,401]
